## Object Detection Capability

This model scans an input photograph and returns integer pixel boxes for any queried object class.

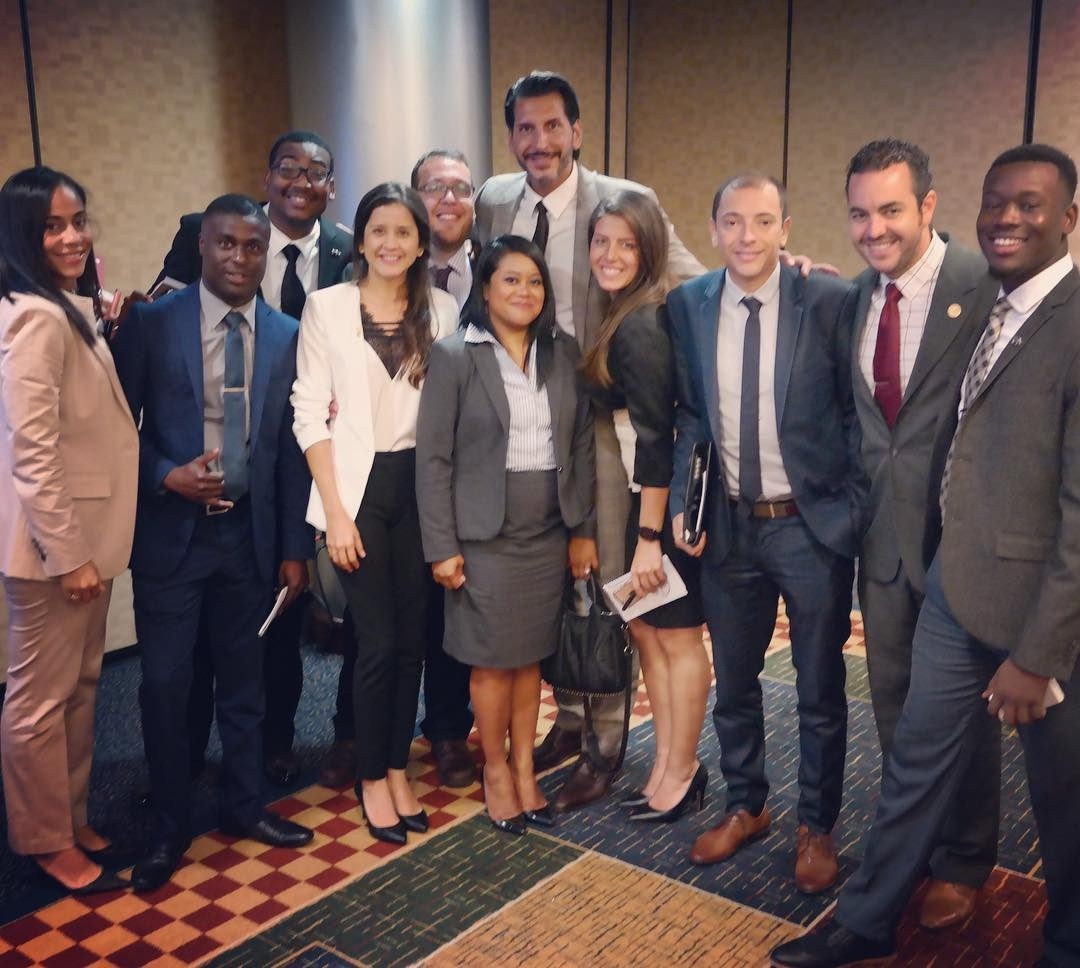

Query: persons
[344,152,708,840]
[767,146,1080,968]
[287,183,462,847]
[844,137,1004,934]
[471,69,837,810]
[0,167,139,895]
[667,169,864,892]
[109,194,317,894]
[144,131,355,792]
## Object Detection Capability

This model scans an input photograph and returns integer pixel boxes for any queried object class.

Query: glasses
[414,182,474,200]
[271,163,329,185]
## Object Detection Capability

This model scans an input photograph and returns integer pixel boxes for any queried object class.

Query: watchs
[638,525,663,543]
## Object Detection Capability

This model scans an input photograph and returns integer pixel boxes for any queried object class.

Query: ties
[431,263,454,291]
[872,282,902,431]
[738,296,762,503]
[533,201,549,253]
[223,313,250,500]
[938,297,1011,527]
[280,243,306,321]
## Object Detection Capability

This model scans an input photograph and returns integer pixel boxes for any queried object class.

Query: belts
[728,498,799,519]
[199,498,231,516]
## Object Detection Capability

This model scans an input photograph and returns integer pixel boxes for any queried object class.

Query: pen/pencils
[621,591,636,612]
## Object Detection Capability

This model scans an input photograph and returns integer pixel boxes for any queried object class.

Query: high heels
[524,806,556,828]
[354,778,407,845]
[480,765,527,836]
[385,768,429,834]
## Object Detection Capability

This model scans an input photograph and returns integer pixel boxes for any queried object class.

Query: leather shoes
[431,735,476,787]
[532,723,975,968]
[319,740,359,787]
[131,833,190,889]
[218,809,313,845]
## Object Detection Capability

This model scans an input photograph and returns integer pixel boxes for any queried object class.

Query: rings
[585,569,591,575]
[70,594,80,601]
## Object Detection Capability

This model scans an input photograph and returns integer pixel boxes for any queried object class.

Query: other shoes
[27,854,133,894]
[79,841,128,867]
